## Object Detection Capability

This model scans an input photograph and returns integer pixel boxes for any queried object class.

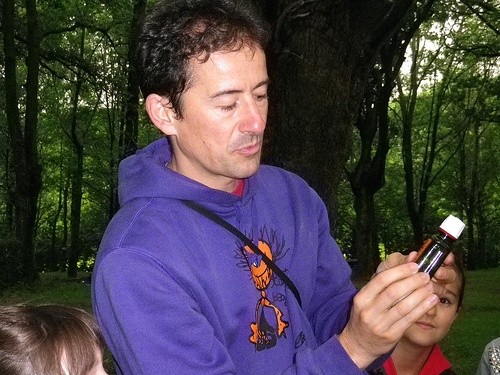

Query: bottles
[414,215,466,283]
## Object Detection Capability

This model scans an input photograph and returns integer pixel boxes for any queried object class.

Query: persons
[92,1,457,375]
[0,302,110,375]
[366,257,465,375]
[475,336,500,375]
